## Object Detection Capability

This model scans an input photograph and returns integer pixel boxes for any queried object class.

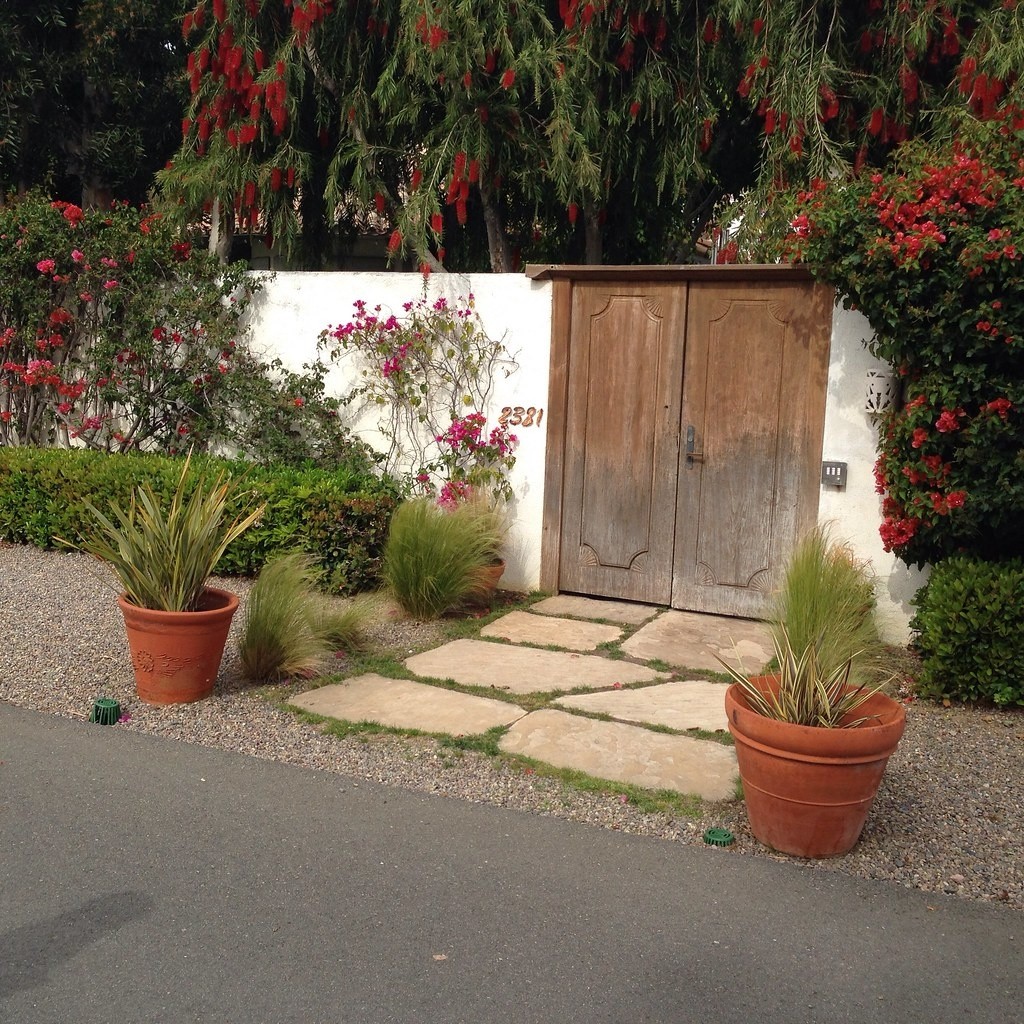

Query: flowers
[416,413,516,512]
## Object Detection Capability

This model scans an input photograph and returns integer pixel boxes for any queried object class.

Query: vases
[463,559,506,604]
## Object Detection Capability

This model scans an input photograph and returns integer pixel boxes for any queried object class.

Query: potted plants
[53,443,268,702]
[702,622,906,857]
